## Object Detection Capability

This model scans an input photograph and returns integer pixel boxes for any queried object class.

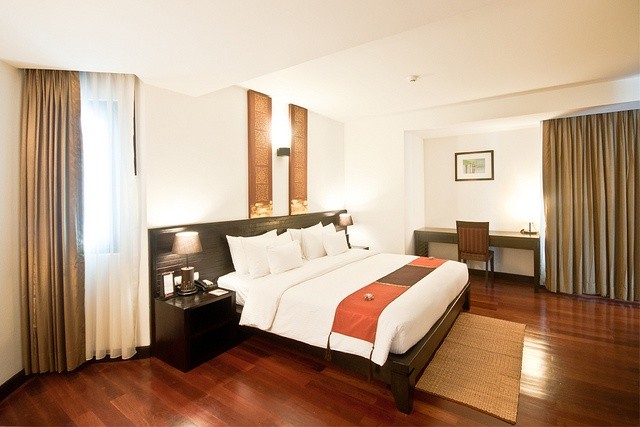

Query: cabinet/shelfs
[156,285,236,372]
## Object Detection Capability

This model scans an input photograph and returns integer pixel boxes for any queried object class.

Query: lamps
[171,231,202,294]
[339,213,354,248]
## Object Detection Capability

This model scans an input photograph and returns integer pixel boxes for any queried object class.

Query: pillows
[270,239,303,271]
[250,232,292,275]
[300,223,336,260]
[321,230,349,255]
[286,221,324,251]
[226,227,277,278]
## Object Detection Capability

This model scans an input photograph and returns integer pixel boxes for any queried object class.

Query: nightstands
[149,208,349,294]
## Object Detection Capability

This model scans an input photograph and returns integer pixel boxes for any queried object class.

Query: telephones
[194,278,217,292]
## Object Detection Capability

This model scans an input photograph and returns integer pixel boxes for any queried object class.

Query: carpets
[414,311,526,423]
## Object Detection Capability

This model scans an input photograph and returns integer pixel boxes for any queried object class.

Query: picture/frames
[455,150,495,180]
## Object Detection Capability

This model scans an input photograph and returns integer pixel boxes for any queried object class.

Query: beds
[219,249,470,414]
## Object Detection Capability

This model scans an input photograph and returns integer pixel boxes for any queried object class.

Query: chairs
[455,220,495,288]
